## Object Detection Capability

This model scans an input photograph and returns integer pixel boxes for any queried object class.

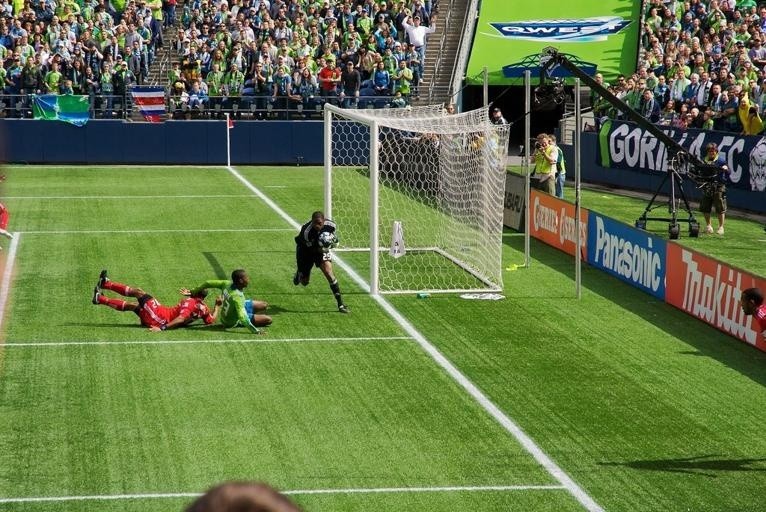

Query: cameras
[534,142,542,149]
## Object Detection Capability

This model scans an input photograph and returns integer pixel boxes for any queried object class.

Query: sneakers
[96,269,110,289]
[91,285,104,305]
[703,224,726,235]
[339,304,351,313]
[293,269,300,286]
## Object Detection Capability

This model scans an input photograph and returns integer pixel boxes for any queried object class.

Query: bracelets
[159,323,167,331]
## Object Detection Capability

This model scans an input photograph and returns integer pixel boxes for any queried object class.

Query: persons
[291,210,350,313]
[696,142,731,235]
[180,269,272,335]
[593,0,766,135]
[424,103,566,199]
[91,269,224,333]
[740,287,766,342]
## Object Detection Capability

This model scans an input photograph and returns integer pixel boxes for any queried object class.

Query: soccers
[319,232,334,247]
